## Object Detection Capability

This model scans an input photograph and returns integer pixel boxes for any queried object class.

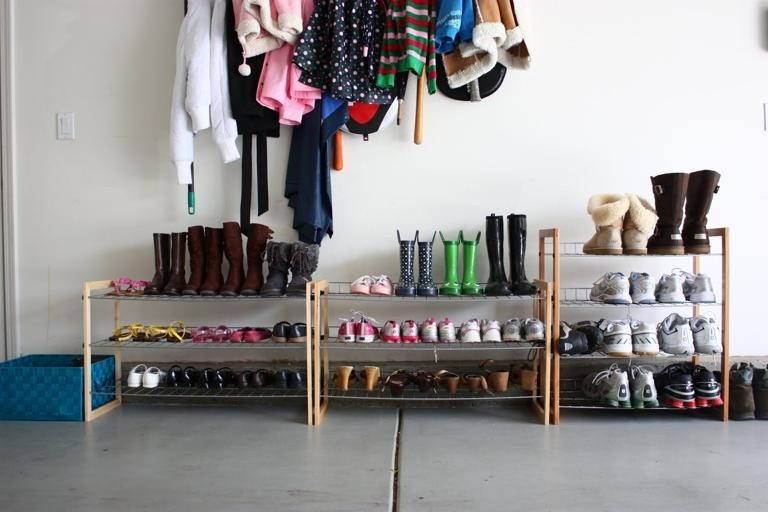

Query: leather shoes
[127,364,307,390]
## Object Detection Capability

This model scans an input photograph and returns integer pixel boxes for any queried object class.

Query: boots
[583,170,721,255]
[107,222,318,296]
[350,214,537,295]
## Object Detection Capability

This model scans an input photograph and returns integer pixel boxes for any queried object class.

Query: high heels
[333,363,539,393]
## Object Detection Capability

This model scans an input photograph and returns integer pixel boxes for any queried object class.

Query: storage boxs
[0,352,116,423]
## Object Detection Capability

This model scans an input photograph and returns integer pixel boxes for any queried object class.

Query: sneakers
[591,268,715,303]
[551,312,723,357]
[581,361,768,419]
[338,317,544,343]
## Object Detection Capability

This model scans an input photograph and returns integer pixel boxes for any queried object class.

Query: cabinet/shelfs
[76,277,324,427]
[308,264,556,434]
[533,222,738,427]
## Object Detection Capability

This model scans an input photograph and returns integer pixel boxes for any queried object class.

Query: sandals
[110,320,314,343]
